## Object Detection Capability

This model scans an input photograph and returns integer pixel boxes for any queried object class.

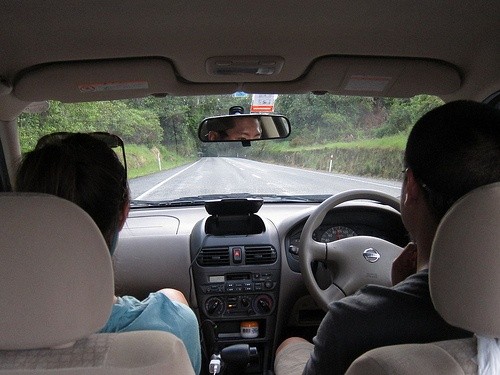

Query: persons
[206,118,262,140]
[273,100,500,375]
[12,130,202,375]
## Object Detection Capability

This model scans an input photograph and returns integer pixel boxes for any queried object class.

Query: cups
[240,321,259,338]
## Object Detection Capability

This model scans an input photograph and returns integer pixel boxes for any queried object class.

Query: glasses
[34,131,127,174]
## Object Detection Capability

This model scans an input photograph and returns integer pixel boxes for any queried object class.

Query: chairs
[343,182,500,375]
[0,192,196,375]
